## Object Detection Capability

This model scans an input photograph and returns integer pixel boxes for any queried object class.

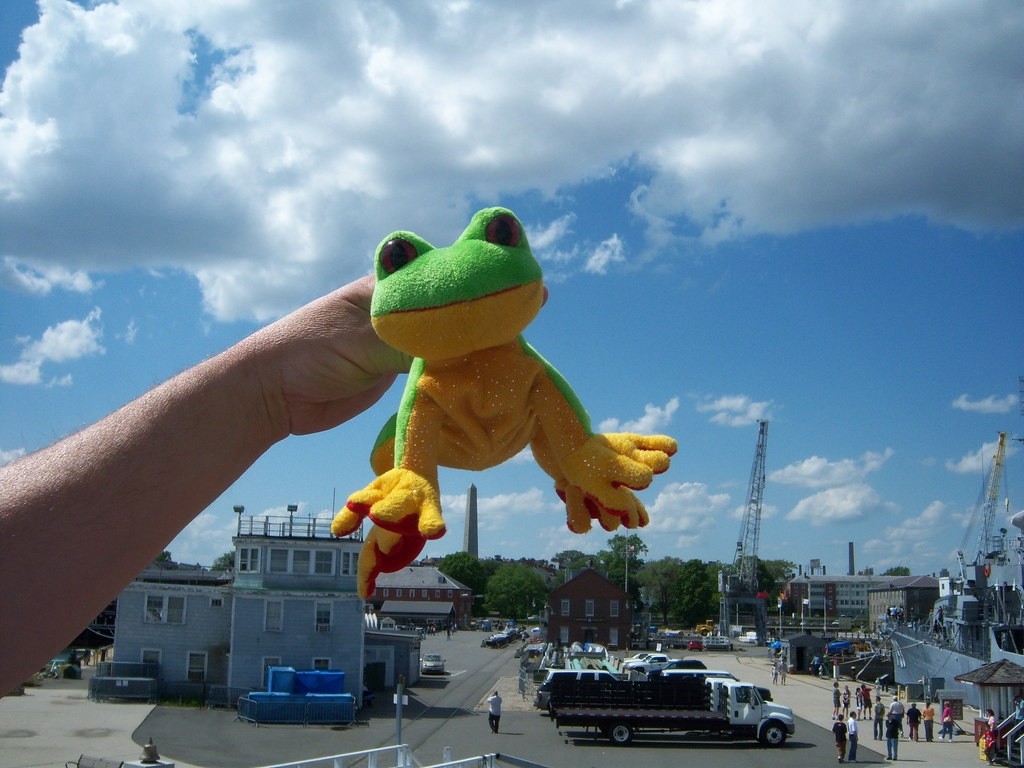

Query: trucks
[550,674,796,749]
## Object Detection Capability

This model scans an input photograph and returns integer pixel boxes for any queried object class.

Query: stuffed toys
[331,206,678,601]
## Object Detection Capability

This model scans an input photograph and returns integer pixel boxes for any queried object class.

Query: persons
[771,658,788,686]
[0,274,414,700]
[886,604,904,627]
[1013,695,1024,738]
[482,619,502,632]
[890,696,905,737]
[847,711,858,762]
[939,701,954,742]
[873,695,885,741]
[811,655,820,676]
[522,632,526,644]
[832,714,847,764]
[831,681,873,721]
[487,690,502,734]
[934,606,943,626]
[906,702,922,742]
[984,708,999,766]
[427,624,455,641]
[885,712,900,761]
[922,701,934,742]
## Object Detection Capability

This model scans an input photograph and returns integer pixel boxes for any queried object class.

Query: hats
[893,696,897,699]
[874,696,881,700]
[886,712,893,716]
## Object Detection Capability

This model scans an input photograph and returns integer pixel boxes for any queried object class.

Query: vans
[659,669,739,683]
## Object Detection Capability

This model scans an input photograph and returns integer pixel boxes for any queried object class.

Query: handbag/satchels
[943,715,951,722]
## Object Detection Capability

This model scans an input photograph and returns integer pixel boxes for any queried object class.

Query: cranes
[953,431,1007,591]
[720,418,769,645]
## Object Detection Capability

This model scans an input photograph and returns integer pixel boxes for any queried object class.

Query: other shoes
[892,758,897,760]
[901,731,904,737]
[869,717,872,720]
[886,757,892,760]
[832,717,838,719]
[939,737,944,740]
[864,716,866,719]
[838,755,845,763]
[949,739,951,742]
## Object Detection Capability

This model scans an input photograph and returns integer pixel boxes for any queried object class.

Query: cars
[623,651,708,679]
[419,653,446,674]
[688,639,703,652]
